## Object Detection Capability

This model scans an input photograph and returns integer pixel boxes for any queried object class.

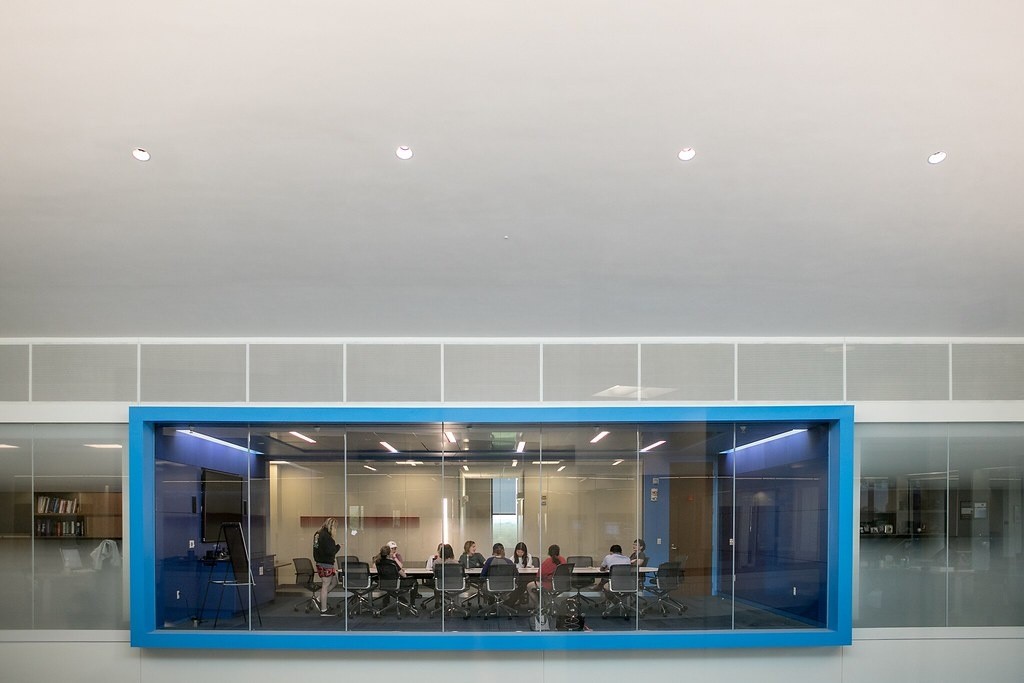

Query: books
[37,496,84,536]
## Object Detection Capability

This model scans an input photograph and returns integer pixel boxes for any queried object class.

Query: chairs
[293,555,686,622]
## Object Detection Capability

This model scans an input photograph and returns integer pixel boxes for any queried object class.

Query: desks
[336,566,659,613]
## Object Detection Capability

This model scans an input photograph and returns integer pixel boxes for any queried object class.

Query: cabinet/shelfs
[32,490,86,538]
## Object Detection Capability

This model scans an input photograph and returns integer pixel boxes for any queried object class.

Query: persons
[527,544,566,602]
[374,541,423,619]
[590,539,646,597]
[481,543,520,594]
[510,542,532,602]
[426,544,459,587]
[310,516,340,618]
[459,540,487,568]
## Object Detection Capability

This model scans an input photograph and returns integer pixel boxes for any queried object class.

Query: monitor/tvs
[201,468,243,543]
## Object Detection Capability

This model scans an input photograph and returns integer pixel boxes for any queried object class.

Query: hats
[386,540,397,548]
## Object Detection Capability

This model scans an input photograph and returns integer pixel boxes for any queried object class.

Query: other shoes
[312,597,321,612]
[320,609,336,616]
